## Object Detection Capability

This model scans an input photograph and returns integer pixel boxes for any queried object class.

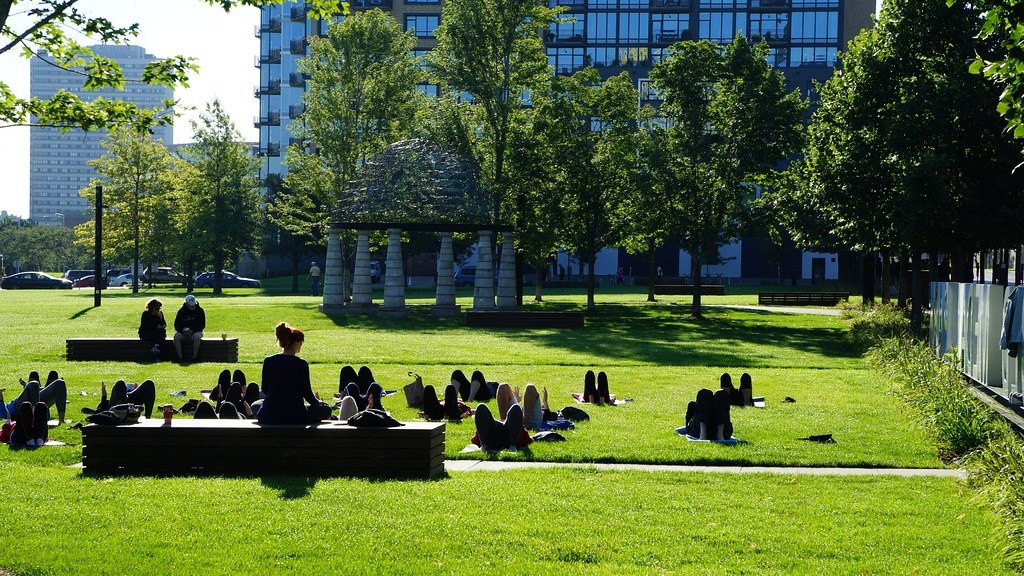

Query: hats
[185,295,198,307]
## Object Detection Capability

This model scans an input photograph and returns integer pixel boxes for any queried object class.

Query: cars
[0,272,74,290]
[109,273,142,287]
[195,270,261,288]
[72,275,97,288]
[454,264,499,287]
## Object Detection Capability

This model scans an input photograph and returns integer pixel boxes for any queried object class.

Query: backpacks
[85,411,119,425]
[108,404,143,422]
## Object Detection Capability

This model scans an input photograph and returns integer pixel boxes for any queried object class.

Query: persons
[617,264,624,285]
[138,300,166,363]
[0,371,68,447]
[657,264,664,282]
[721,373,754,407]
[310,262,320,295]
[572,370,615,406]
[921,258,951,310]
[421,369,559,451]
[686,389,734,443]
[999,285,1024,359]
[194,368,265,419]
[890,257,901,273]
[261,324,332,422]
[95,380,155,416]
[174,295,206,362]
[559,264,565,280]
[335,364,386,422]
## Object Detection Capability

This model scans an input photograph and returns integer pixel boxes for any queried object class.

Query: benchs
[81,419,446,478]
[465,311,585,328]
[654,285,725,295]
[66,338,239,363]
[545,281,599,288]
[759,291,849,306]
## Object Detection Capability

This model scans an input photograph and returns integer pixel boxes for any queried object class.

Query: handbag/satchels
[403,371,425,406]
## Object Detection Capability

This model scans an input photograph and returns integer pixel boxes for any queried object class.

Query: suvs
[106,268,130,285]
[143,267,189,284]
[65,269,95,282]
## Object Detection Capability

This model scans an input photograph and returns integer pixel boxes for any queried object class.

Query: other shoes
[599,400,606,406]
[27,439,36,445]
[36,438,44,445]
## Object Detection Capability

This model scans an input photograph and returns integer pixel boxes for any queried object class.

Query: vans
[370,260,381,284]
